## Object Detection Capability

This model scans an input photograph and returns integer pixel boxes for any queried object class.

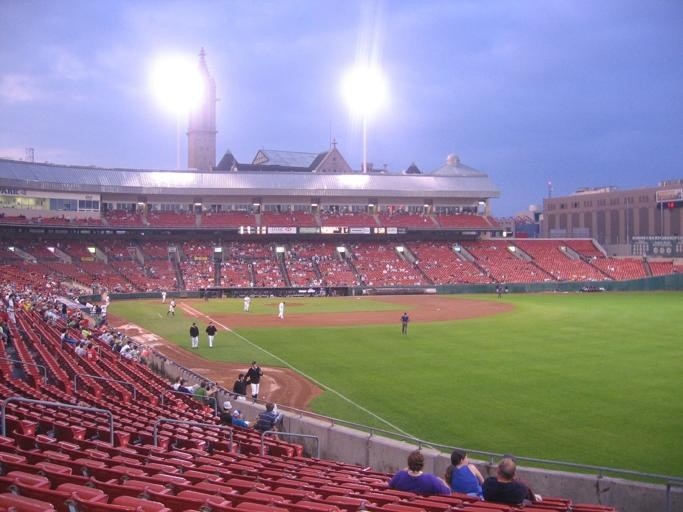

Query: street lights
[345,56,382,174]
[151,48,199,170]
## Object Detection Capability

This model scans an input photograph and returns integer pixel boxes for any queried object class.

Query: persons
[481,457,542,504]
[205,322,217,348]
[443,450,484,499]
[189,323,199,348]
[399,311,408,332]
[382,451,449,494]
[166,299,176,317]
[151,239,417,296]
[160,290,166,303]
[243,294,250,311]
[173,358,288,438]
[0,274,151,364]
[278,302,284,319]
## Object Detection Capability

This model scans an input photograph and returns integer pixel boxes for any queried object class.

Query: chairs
[0,296,264,434]
[1,434,617,512]
[1,193,682,295]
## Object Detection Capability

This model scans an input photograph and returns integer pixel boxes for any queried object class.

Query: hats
[234,409,241,418]
[224,402,233,410]
